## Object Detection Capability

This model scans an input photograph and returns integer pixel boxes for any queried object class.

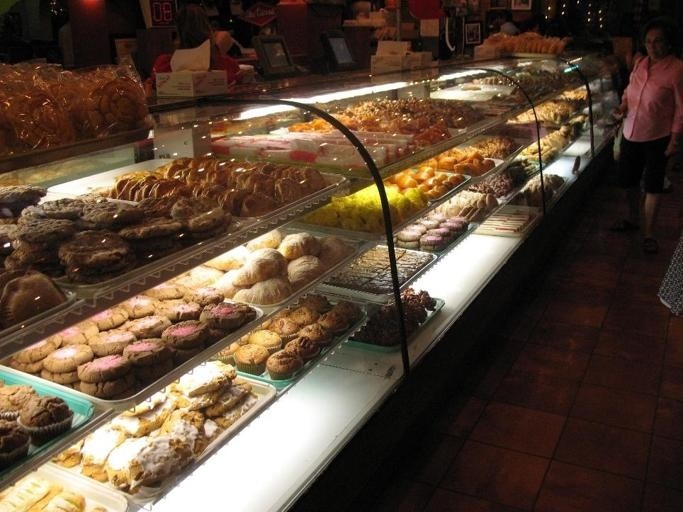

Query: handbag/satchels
[612,122,623,162]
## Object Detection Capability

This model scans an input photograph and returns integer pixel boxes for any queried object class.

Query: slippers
[610,219,640,231]
[642,237,659,253]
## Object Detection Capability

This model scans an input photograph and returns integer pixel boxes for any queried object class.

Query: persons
[148,7,241,91]
[611,25,682,254]
[523,21,540,33]
[498,10,521,35]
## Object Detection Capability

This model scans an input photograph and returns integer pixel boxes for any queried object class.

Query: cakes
[1,31,588,512]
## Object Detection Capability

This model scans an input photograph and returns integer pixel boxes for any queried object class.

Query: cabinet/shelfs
[453,47,621,212]
[152,67,545,379]
[0,103,407,512]
